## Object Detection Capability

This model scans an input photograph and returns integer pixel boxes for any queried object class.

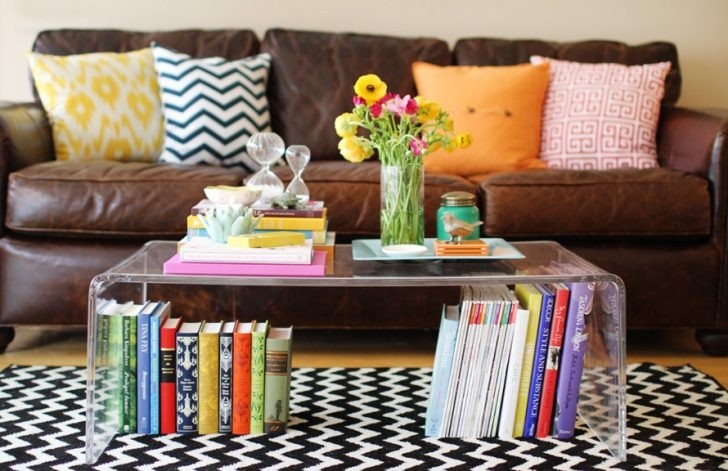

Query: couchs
[0,27,728,356]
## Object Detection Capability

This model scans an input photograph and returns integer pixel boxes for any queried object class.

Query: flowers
[335,74,474,242]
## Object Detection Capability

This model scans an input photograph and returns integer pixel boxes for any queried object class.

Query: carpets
[0,364,728,471]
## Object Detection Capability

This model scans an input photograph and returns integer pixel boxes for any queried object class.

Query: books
[423,259,596,439]
[121,299,294,435]
[97,298,134,367]
[163,199,336,276]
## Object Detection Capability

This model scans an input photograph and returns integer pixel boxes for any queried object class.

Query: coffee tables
[85,241,628,462]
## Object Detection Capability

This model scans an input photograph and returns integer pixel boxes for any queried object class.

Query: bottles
[436,191,480,240]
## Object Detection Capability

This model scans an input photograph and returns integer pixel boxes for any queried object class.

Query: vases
[378,164,425,245]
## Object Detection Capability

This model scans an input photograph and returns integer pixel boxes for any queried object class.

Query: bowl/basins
[203,185,263,207]
[382,244,427,257]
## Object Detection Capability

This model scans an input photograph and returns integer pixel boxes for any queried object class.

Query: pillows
[530,54,672,170]
[411,60,552,175]
[21,46,165,161]
[150,40,274,166]
